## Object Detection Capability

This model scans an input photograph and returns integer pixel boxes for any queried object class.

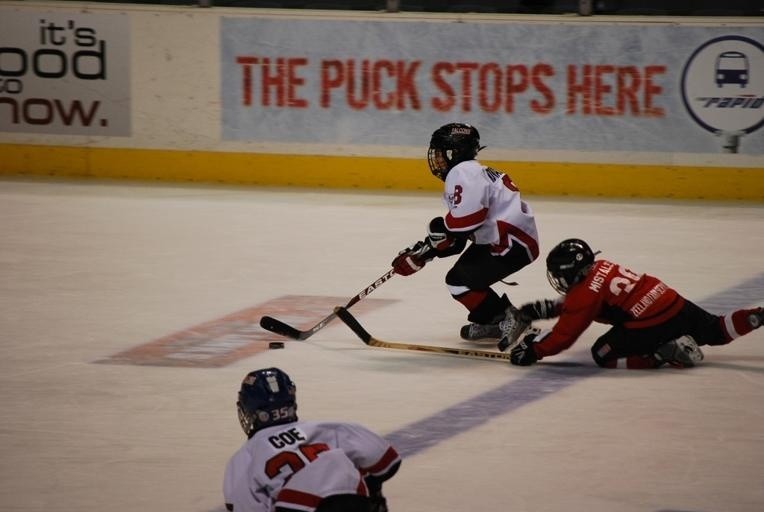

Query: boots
[499,305,530,349]
[460,322,501,339]
[659,341,693,367]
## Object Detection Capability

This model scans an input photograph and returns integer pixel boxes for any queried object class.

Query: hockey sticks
[336,305,512,360]
[259,238,430,343]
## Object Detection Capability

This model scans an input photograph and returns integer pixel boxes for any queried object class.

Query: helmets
[546,238,595,295]
[236,367,297,437]
[427,123,480,181]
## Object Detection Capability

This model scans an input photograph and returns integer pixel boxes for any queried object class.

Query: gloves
[392,240,433,275]
[428,216,467,257]
[518,300,556,322]
[510,334,541,367]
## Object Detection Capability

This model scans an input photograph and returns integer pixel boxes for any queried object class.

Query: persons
[511,238,764,370]
[391,123,540,354]
[221,367,402,512]
